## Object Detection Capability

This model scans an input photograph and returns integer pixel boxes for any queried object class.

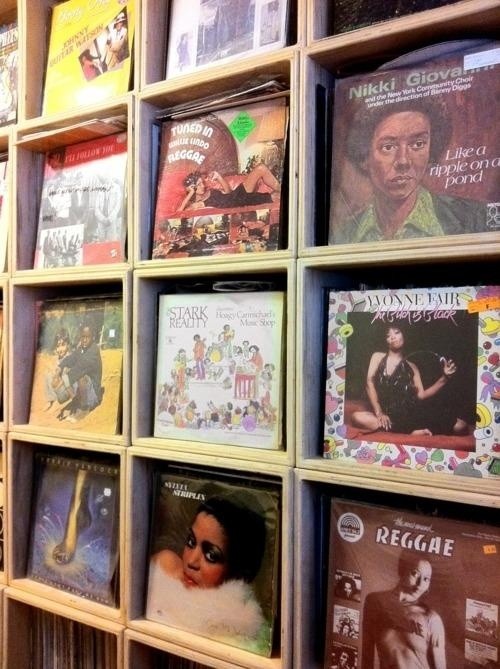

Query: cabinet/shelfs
[292,1,500,669]
[1,2,144,669]
[123,0,310,669]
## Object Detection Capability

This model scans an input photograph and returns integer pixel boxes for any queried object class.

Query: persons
[39,167,123,268]
[350,325,469,437]
[104,12,127,64]
[158,160,281,255]
[44,329,75,412]
[158,323,276,432]
[81,54,100,81]
[176,33,189,70]
[53,323,103,423]
[326,548,496,669]
[146,497,267,642]
[334,96,486,244]
